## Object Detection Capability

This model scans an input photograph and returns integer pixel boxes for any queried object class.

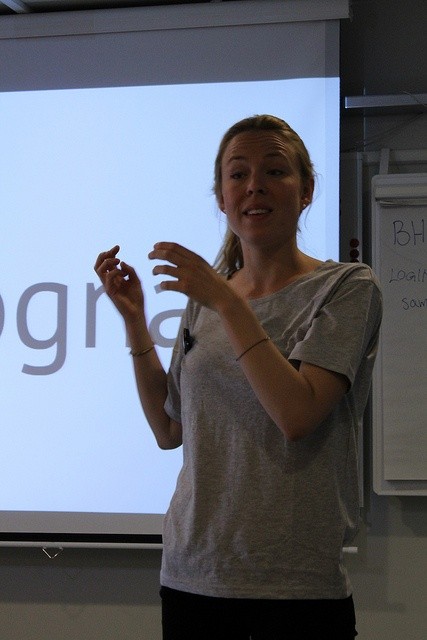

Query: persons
[92,109,385,640]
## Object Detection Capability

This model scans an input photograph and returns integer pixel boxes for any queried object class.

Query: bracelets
[126,340,156,357]
[231,333,274,363]
